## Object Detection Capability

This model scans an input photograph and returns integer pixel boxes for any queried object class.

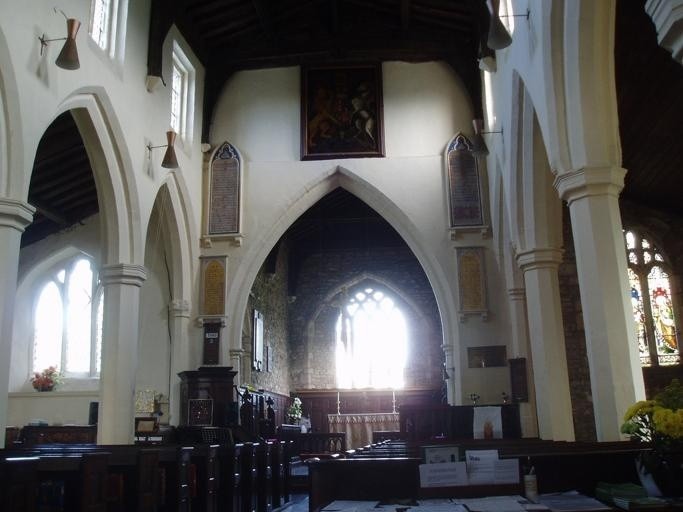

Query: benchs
[4,432,295,511]
[300,432,655,512]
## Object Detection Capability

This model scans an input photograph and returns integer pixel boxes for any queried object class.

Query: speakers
[202,144,211,153]
[478,57,496,73]
[509,357,528,402]
[146,76,164,94]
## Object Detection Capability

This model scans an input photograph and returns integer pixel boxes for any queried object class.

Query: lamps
[149,129,184,169]
[36,18,81,71]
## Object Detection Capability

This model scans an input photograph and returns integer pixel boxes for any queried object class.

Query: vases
[37,382,55,393]
[636,441,682,500]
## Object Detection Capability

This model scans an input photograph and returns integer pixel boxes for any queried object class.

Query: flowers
[27,362,65,387]
[619,377,683,449]
[287,395,308,420]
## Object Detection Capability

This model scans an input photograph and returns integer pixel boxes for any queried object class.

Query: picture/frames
[296,59,390,161]
